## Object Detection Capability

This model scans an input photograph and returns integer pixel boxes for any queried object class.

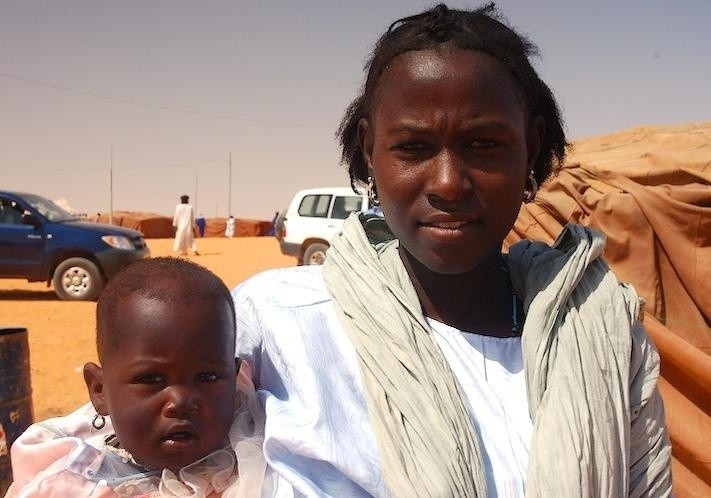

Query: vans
[275,187,381,267]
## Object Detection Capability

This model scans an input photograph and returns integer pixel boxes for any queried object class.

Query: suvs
[0,189,151,302]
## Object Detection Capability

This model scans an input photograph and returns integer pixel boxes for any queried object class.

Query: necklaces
[504,262,520,339]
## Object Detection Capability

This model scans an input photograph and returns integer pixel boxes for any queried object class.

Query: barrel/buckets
[0,324,36,497]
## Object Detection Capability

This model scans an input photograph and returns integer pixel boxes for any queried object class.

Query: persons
[2,254,282,497]
[223,2,677,496]
[268,211,278,234]
[94,212,101,223]
[195,212,208,237]
[224,214,236,239]
[170,193,201,256]
